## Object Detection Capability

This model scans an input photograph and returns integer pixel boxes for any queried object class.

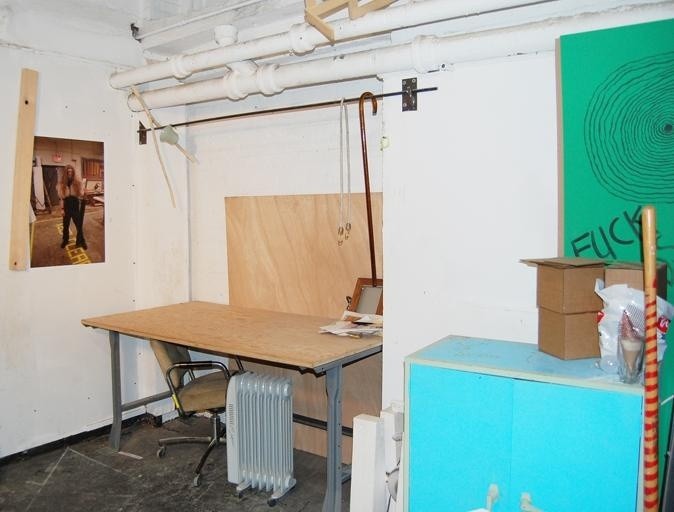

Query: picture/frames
[347,278,383,315]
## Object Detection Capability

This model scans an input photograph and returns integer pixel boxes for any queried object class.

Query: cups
[616,333,646,383]
[159,126,178,145]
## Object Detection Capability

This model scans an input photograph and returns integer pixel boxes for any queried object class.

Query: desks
[79,298,382,511]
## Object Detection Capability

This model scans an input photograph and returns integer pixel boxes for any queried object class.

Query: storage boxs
[604,262,669,307]
[537,303,602,363]
[532,265,609,316]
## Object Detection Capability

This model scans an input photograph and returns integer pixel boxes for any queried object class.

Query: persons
[60,165,88,250]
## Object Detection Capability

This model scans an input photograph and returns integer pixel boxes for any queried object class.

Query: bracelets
[61,208,65,209]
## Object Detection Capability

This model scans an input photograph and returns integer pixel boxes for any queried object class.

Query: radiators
[222,370,297,507]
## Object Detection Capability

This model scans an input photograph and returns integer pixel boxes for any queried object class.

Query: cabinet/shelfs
[397,333,659,512]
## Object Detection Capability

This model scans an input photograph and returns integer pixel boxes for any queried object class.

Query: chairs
[145,332,258,486]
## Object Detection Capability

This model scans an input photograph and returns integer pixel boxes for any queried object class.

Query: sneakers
[82,239,88,250]
[61,239,69,249]
